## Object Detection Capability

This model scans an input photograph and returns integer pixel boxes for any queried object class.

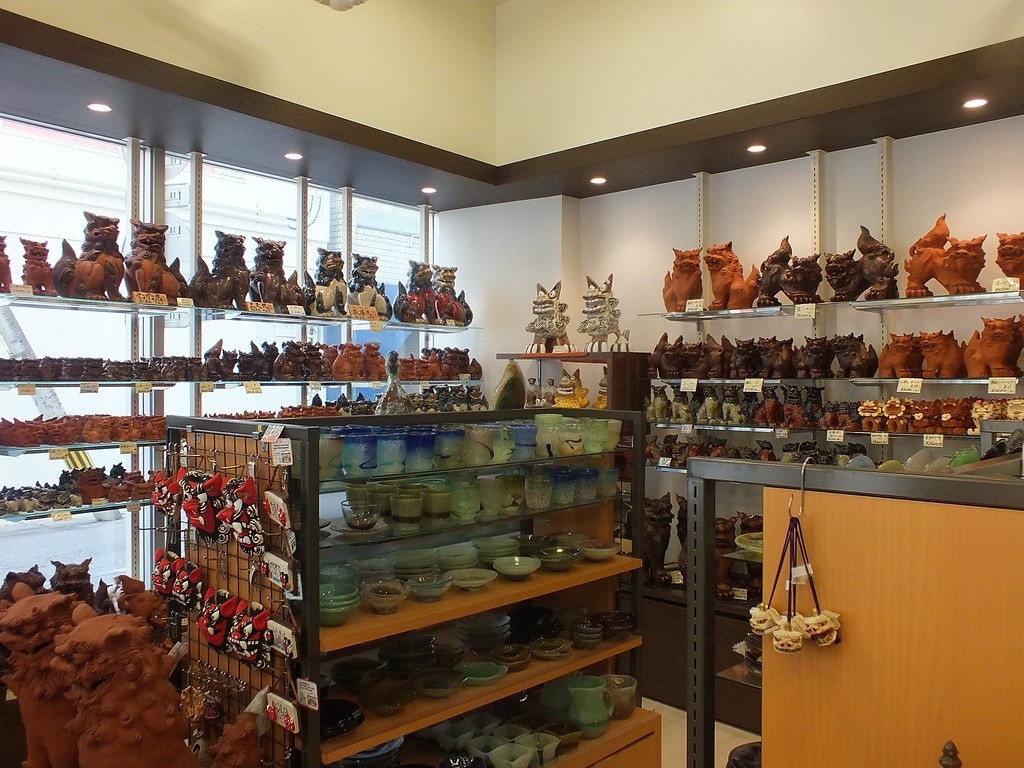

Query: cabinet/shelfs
[1,294,1024,768]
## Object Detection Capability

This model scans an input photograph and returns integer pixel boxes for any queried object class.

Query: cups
[318,411,620,534]
[417,703,582,768]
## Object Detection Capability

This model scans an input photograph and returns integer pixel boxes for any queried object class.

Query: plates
[314,531,634,741]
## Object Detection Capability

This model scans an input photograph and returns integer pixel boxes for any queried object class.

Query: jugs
[531,669,615,739]
